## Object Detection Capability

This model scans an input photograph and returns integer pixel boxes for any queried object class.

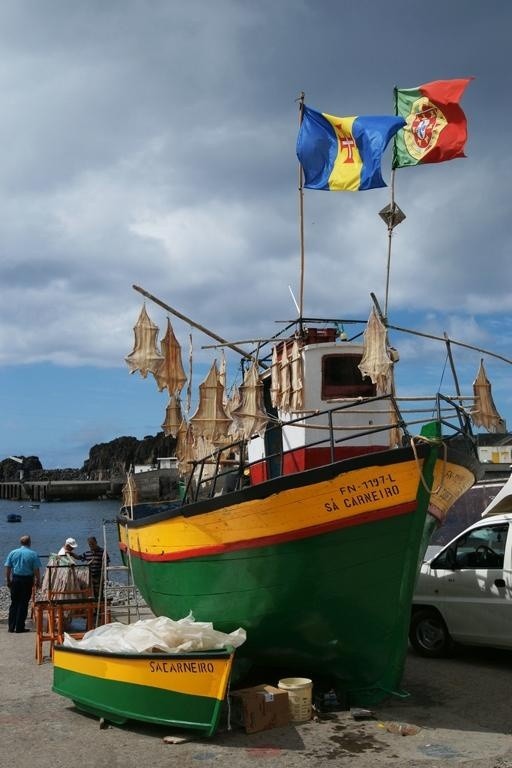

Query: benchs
[33,595,114,663]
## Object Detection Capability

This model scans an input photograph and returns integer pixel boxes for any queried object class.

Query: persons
[57,536,79,563]
[4,534,41,632]
[64,536,111,598]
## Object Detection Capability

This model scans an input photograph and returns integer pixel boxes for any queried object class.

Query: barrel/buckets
[277,677,313,724]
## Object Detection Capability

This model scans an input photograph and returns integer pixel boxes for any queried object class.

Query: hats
[66,538,79,548]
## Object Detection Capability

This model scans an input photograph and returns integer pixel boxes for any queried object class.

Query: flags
[297,104,407,191]
[393,76,470,170]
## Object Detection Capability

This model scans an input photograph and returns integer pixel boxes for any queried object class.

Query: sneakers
[7,629,30,633]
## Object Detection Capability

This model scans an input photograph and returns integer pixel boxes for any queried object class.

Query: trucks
[407,463,512,662]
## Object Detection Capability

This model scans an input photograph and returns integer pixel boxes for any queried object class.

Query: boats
[6,514,22,522]
[48,642,237,738]
[111,308,490,710]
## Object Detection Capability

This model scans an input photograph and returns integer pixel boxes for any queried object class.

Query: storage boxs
[223,684,288,734]
